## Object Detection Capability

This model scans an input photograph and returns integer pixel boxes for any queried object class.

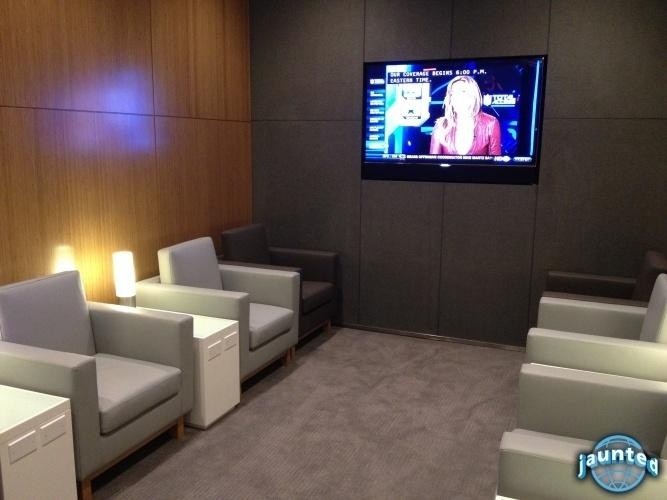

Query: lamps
[113,251,135,308]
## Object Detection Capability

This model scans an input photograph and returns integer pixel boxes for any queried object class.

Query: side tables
[0,384,78,499]
[133,304,242,428]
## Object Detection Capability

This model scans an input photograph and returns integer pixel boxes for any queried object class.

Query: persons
[430,75,502,155]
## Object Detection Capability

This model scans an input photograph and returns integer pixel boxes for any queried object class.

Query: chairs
[495,364,667,499]
[127,236,301,385]
[213,224,344,369]
[539,253,664,305]
[520,273,667,380]
[0,270,196,500]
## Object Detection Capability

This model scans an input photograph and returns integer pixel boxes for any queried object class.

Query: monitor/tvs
[360,53,548,185]
